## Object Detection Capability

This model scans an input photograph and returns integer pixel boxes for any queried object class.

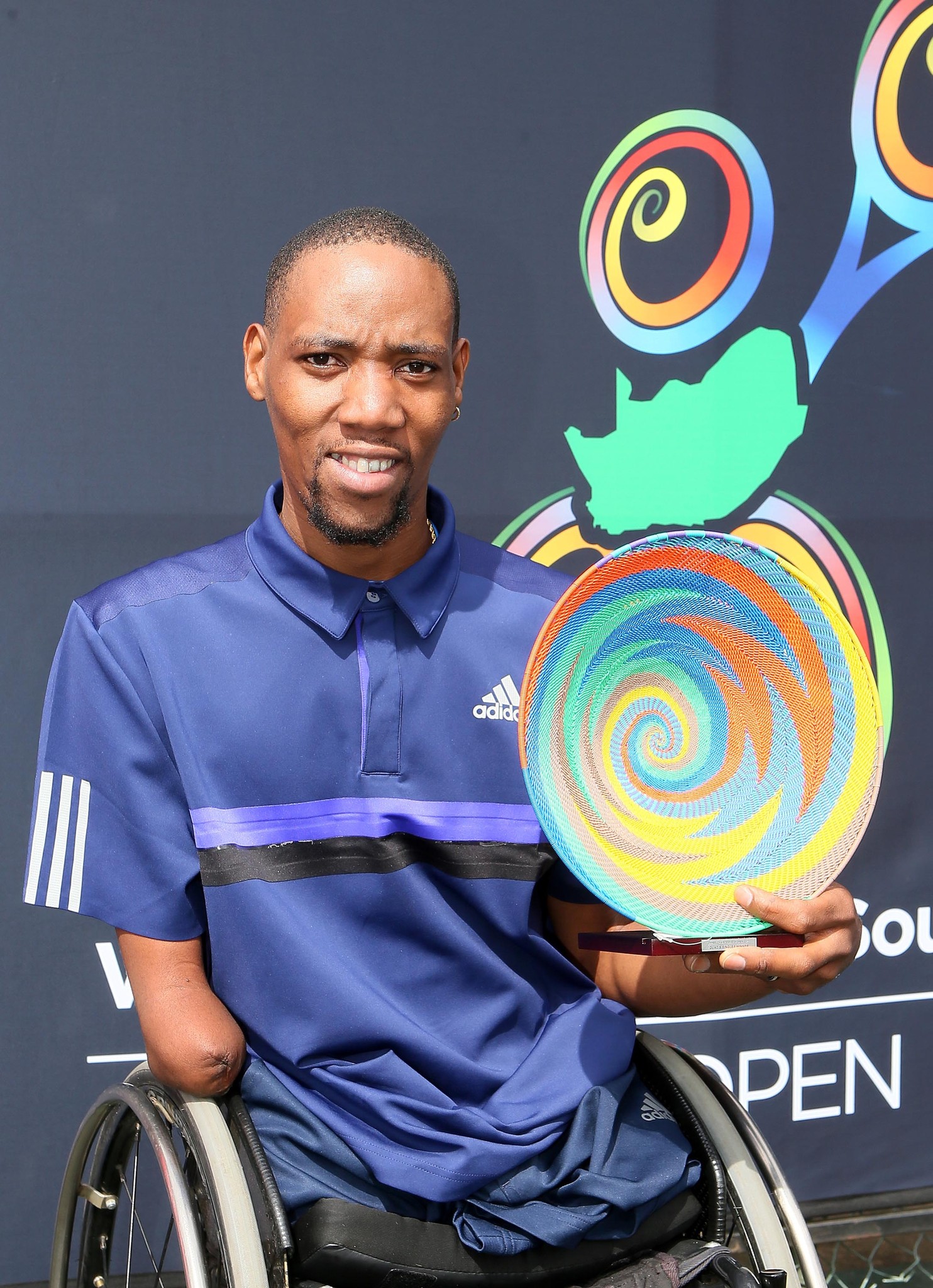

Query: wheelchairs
[52,1017,826,1288]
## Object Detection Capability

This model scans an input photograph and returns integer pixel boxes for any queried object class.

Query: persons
[25,209,861,1288]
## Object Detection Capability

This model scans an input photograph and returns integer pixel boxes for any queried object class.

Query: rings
[769,976,779,982]
